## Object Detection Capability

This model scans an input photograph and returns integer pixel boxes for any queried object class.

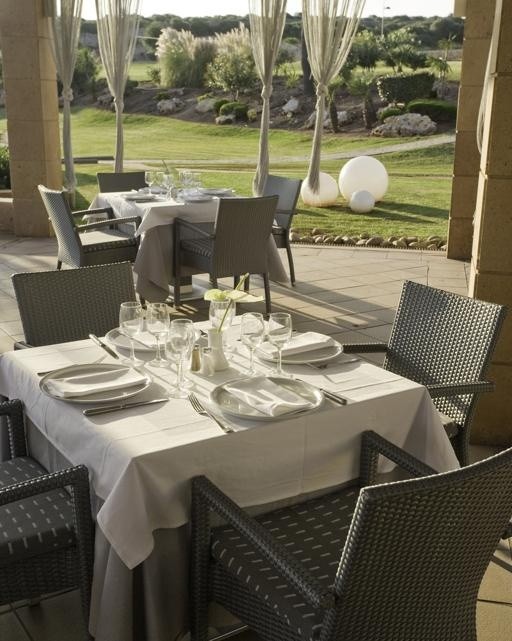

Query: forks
[188,393,233,435]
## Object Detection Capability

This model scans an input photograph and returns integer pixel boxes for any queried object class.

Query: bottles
[191,345,216,379]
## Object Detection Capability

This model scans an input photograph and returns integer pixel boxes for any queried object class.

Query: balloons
[301,172,339,207]
[348,190,376,213]
[339,155,388,203]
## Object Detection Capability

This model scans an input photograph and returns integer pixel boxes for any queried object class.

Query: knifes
[89,331,119,359]
[81,397,170,415]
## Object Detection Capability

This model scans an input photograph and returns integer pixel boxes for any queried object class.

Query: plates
[38,362,152,404]
[120,188,233,202]
[249,333,342,364]
[105,319,203,353]
[210,375,325,422]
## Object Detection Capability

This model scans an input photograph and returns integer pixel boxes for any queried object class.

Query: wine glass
[143,168,201,202]
[118,296,291,400]
